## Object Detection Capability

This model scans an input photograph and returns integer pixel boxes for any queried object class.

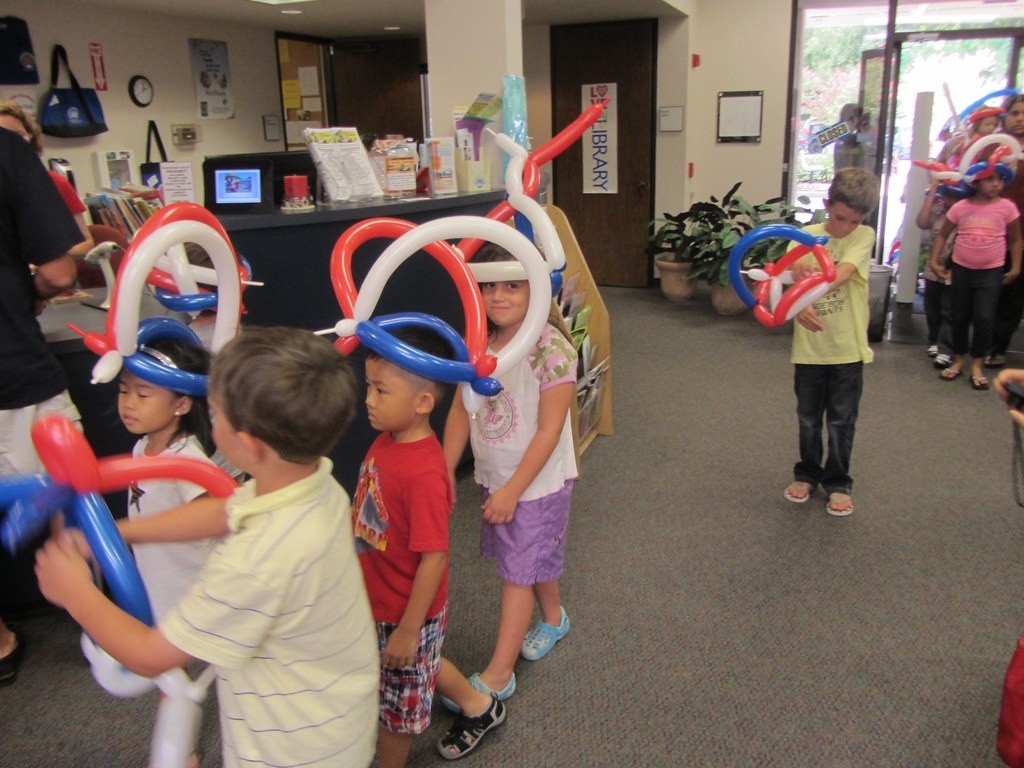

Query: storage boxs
[372,155,417,197]
[96,149,136,189]
[455,147,492,193]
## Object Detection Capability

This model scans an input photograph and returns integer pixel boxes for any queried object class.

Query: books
[86,190,160,240]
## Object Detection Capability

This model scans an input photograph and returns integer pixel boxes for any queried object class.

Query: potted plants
[644,181,827,320]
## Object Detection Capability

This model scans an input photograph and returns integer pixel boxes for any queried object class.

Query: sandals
[442,672,517,712]
[521,605,570,661]
[437,693,507,760]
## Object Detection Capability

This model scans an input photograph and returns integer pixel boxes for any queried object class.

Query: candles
[284,174,309,201]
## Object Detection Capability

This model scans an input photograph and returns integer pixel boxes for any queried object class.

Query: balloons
[728,224,837,328]
[911,89,1018,198]
[314,99,611,420]
[66,202,252,396]
[0,413,239,768]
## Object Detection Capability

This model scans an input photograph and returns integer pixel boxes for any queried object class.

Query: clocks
[128,75,153,107]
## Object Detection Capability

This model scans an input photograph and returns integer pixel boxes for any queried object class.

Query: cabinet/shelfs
[504,204,615,481]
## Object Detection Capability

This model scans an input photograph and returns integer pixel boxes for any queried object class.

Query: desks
[36,284,193,355]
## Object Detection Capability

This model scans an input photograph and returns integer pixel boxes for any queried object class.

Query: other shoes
[927,345,939,356]
[933,353,954,366]
[985,354,1004,367]
[0,622,22,679]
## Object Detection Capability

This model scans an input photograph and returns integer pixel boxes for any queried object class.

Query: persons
[0,102,94,271]
[994,369,1024,427]
[350,328,507,768]
[34,328,382,768]
[0,128,103,680]
[227,178,240,192]
[116,339,217,768]
[784,168,879,516]
[443,243,580,713]
[916,95,1024,390]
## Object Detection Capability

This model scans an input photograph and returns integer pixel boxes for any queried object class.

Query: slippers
[826,494,853,516]
[784,481,813,503]
[940,368,963,380]
[969,376,988,389]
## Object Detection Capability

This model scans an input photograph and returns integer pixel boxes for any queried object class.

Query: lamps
[81,240,120,313]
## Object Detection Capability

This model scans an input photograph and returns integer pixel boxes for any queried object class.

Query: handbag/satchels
[37,44,109,137]
[140,120,173,190]
